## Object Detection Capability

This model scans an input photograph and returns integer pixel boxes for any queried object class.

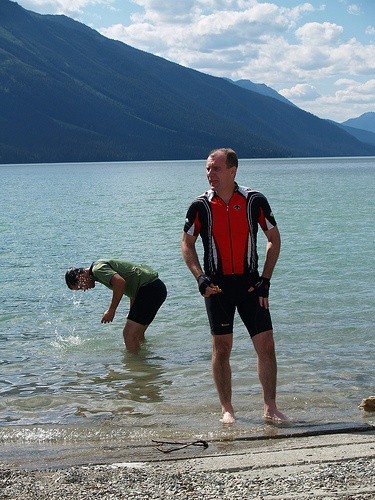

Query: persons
[65,258,167,354]
[180,148,289,424]
